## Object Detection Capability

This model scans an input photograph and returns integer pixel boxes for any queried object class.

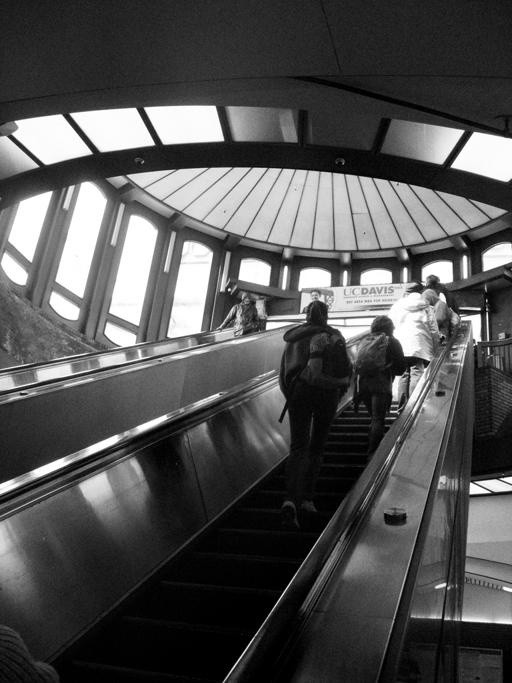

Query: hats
[406,282,425,293]
[241,292,250,301]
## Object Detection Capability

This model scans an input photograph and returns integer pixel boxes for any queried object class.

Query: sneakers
[281,500,300,530]
[301,500,317,512]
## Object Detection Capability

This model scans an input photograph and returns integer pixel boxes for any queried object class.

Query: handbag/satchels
[301,333,350,402]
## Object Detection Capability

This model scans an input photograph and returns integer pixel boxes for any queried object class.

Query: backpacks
[355,331,389,382]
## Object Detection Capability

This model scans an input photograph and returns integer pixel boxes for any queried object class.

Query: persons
[255,296,268,331]
[352,274,458,458]
[301,291,321,313]
[278,301,351,530]
[217,292,260,339]
